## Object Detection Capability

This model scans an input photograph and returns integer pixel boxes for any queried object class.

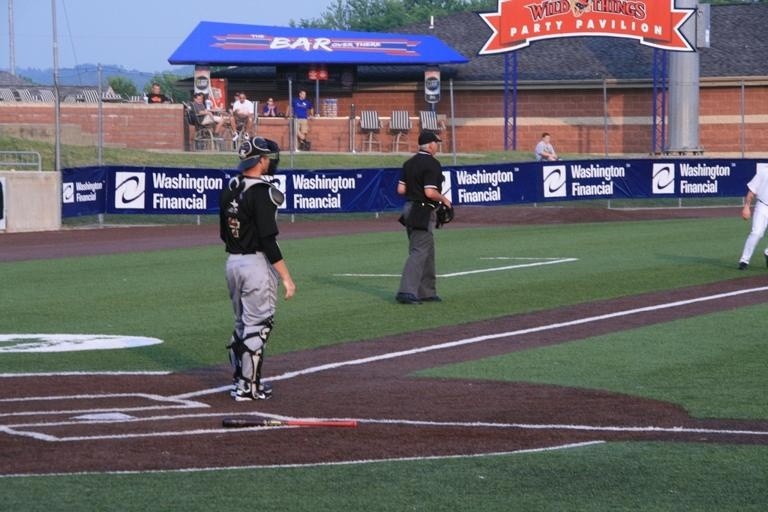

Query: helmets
[238,135,280,177]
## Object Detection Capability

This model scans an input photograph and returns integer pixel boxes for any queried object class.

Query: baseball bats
[223,417,357,428]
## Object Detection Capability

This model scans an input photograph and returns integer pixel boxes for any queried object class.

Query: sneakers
[235,382,273,403]
[737,262,747,270]
[231,379,272,398]
[231,131,238,141]
[244,132,248,139]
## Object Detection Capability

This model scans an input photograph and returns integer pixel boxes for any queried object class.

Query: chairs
[182,99,260,154]
[1,84,102,104]
[359,110,443,153]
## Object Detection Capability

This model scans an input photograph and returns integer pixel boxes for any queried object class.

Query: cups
[143,97,149,104]
[205,100,211,110]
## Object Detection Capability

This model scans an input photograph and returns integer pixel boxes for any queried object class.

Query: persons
[144,83,171,104]
[396,130,452,305]
[262,97,277,117]
[285,90,314,151]
[535,132,557,161]
[219,137,296,400]
[193,90,255,141]
[738,166,768,270]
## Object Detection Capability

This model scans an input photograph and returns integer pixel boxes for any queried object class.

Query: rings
[448,202,450,205]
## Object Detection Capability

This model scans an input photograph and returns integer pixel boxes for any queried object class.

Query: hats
[418,129,443,145]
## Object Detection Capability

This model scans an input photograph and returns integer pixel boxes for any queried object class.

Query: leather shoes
[396,293,423,305]
[421,296,444,303]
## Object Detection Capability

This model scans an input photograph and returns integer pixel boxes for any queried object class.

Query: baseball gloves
[435,204,454,229]
